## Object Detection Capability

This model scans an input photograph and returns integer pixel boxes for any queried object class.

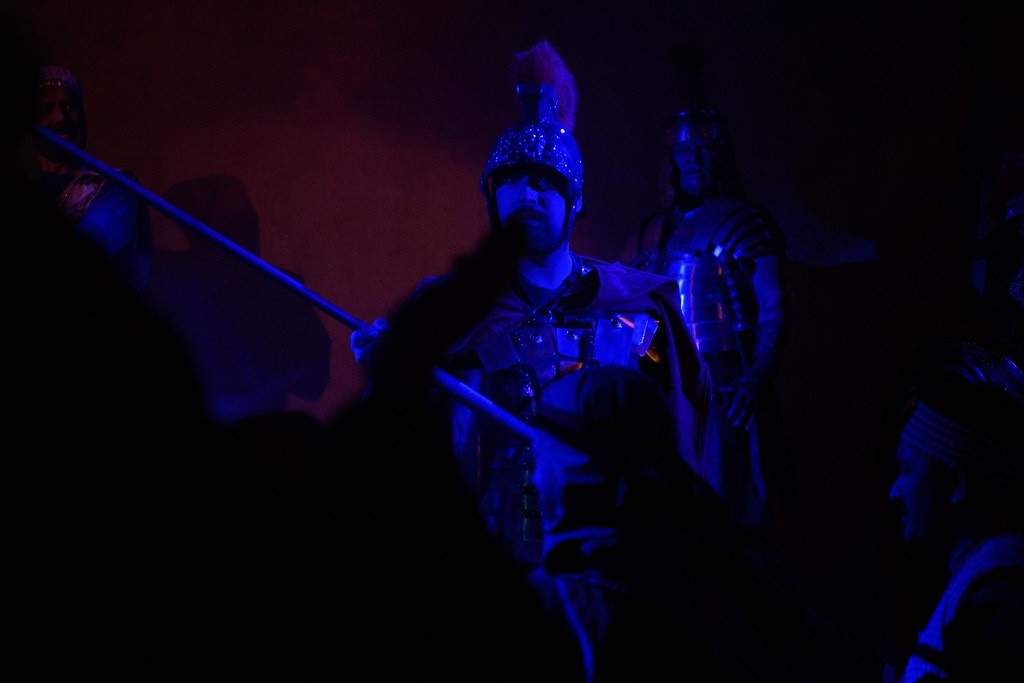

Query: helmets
[661,106,735,193]
[480,46,584,245]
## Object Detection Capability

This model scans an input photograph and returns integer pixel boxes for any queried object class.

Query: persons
[634,106,787,539]
[16,68,148,305]
[349,120,713,560]
[883,339,1024,683]
